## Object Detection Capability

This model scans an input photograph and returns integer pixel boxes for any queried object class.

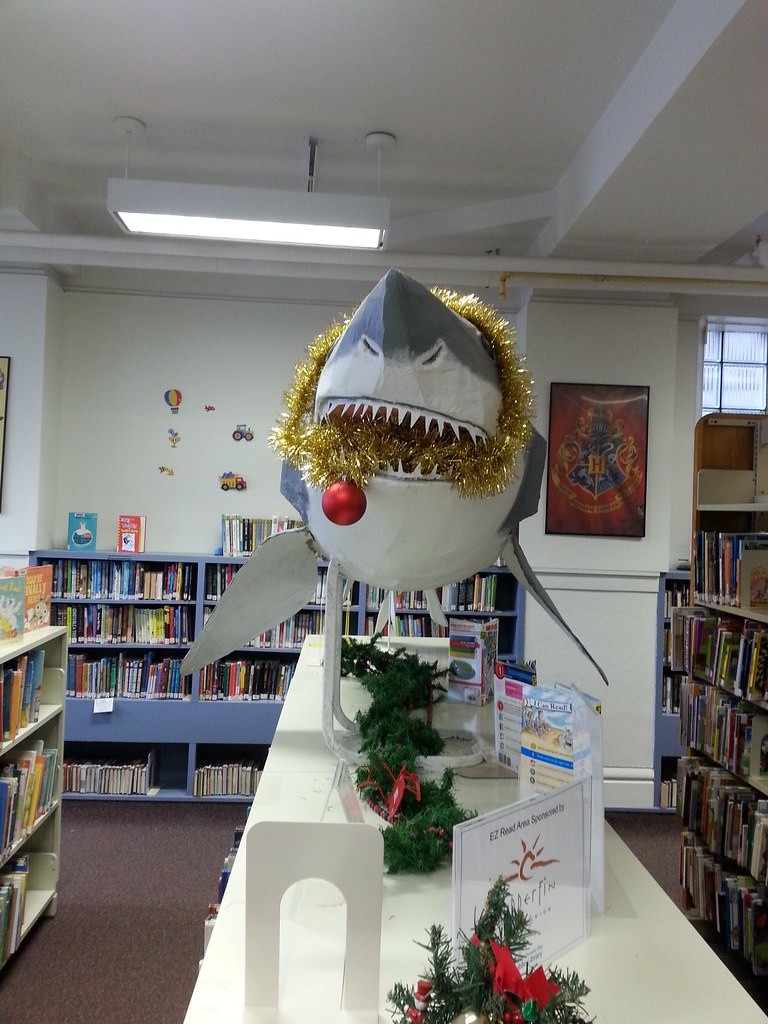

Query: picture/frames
[0,356,11,515]
[544,383,650,538]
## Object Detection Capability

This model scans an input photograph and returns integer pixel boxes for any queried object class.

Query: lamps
[105,117,399,252]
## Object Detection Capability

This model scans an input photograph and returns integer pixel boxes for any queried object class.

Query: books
[493,657,540,778]
[657,518,768,987]
[31,504,506,802]
[197,802,257,983]
[0,559,62,970]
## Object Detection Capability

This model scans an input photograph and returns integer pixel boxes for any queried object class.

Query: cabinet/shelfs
[1,625,68,974]
[29,550,527,804]
[680,413,768,1016]
[653,573,691,809]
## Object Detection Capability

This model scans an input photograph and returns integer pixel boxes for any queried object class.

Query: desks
[182,630,768,1024]
